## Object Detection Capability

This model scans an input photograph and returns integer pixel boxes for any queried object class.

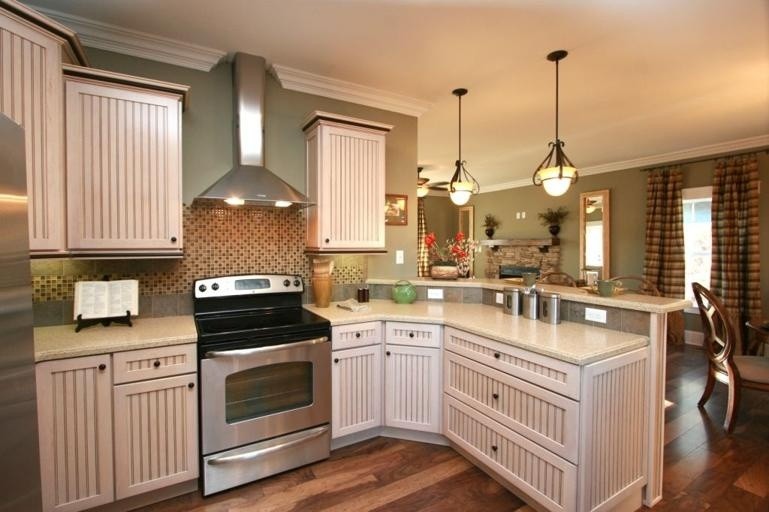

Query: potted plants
[537,206,568,235]
[483,214,499,240]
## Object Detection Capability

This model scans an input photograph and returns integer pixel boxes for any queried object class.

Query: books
[73,279,140,318]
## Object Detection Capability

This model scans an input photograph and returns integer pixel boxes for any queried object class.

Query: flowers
[425,232,466,263]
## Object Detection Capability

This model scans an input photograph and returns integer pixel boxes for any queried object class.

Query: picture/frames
[384,194,408,226]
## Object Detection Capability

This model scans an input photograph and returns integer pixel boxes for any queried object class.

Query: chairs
[607,275,660,296]
[537,272,577,288]
[692,282,769,434]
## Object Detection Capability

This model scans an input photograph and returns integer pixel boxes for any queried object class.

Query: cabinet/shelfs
[299,109,395,256]
[110,342,200,511]
[442,324,648,512]
[384,320,442,446]
[331,320,384,451]
[34,351,115,511]
[0,6,66,258]
[63,61,191,262]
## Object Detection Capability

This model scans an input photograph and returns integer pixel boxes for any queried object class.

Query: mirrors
[579,189,611,286]
[458,204,474,279]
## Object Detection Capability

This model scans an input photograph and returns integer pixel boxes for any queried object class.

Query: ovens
[201,337,331,497]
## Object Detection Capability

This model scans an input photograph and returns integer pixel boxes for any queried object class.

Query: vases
[431,261,459,279]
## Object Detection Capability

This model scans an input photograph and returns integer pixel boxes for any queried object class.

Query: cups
[522,273,541,288]
[520,292,539,320]
[592,278,616,297]
[310,274,330,309]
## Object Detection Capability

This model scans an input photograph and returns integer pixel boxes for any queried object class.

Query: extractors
[191,51,317,209]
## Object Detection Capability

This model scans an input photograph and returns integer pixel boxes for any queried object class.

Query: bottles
[363,287,370,302]
[392,280,418,304]
[358,288,363,302]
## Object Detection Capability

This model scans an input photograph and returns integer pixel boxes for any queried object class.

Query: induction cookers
[193,305,331,348]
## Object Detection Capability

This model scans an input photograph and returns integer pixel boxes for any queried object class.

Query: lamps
[533,50,579,197]
[586,197,597,214]
[417,166,430,198]
[448,88,480,206]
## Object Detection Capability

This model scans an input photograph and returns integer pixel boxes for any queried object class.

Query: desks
[744,317,769,356]
